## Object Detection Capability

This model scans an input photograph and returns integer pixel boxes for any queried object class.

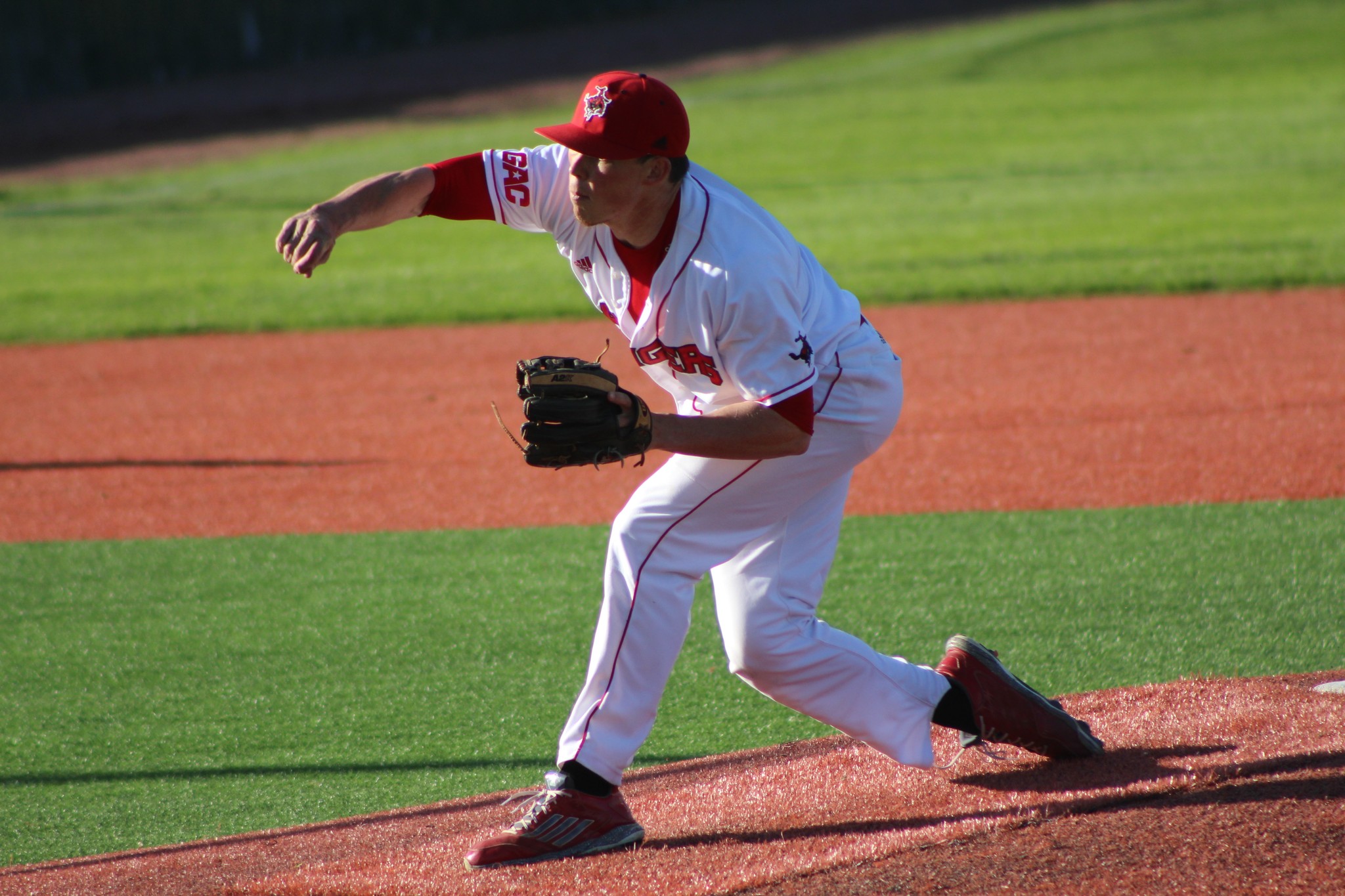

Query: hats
[534,71,690,160]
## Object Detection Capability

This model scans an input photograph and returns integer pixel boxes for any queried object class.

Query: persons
[274,71,1105,870]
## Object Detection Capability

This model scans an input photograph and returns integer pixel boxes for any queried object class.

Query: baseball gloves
[514,354,654,470]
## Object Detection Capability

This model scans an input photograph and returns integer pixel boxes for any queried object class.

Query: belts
[860,315,866,326]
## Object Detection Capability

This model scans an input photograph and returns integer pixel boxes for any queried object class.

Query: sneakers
[465,788,645,867]
[934,635,1105,761]
